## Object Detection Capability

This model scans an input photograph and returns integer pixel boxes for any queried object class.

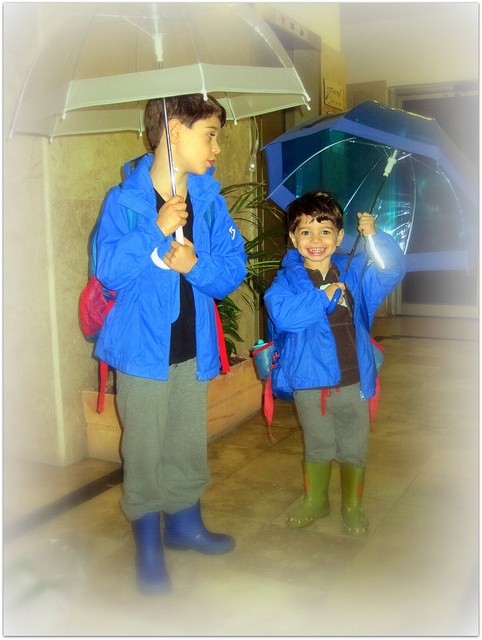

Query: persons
[251,191,409,537]
[79,93,247,611]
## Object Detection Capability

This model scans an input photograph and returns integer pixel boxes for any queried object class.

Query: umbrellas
[11,4,312,270]
[260,99,479,313]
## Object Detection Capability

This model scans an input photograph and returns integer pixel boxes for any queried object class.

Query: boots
[339,461,369,537]
[163,500,235,555]
[286,460,332,529]
[131,511,173,598]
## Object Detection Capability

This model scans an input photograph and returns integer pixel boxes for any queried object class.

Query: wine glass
[251,339,275,381]
